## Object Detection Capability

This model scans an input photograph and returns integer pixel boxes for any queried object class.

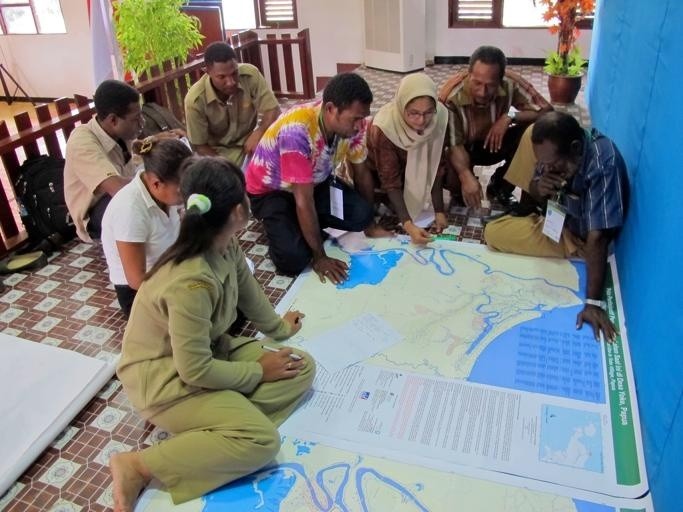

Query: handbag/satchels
[14,155,78,245]
[138,103,187,136]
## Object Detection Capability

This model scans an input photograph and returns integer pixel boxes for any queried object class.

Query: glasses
[405,105,438,120]
[536,154,565,174]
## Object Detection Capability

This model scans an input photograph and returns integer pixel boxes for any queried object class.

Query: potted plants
[539,0,593,106]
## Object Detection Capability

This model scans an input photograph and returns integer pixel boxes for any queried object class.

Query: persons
[484,111,629,344]
[367,72,450,244]
[63,79,187,243]
[244,73,397,285]
[438,46,554,210]
[184,42,282,166]
[101,138,255,336]
[109,156,316,512]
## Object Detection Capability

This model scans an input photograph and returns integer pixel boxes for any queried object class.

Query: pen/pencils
[261,345,301,360]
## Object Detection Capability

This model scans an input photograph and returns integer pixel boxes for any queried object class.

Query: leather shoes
[486,174,518,208]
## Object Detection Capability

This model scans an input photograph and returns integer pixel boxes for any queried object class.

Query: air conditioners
[360,0,426,73]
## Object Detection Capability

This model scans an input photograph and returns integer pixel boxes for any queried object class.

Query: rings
[288,362,292,369]
[324,270,330,275]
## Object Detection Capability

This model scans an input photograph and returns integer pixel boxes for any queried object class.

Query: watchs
[584,298,607,312]
[508,112,516,123]
